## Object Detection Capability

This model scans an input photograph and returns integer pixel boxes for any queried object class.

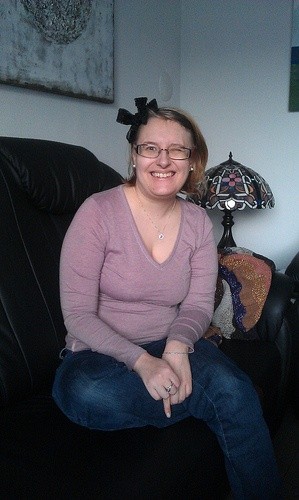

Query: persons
[53,96,287,500]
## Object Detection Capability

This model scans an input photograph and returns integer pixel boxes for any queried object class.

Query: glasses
[132,144,192,160]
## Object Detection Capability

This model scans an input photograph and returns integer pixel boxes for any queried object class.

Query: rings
[165,383,173,392]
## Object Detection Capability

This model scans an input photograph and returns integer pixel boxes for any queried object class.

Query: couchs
[0,137,299,500]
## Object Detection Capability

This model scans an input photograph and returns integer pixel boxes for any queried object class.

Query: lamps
[186,151,275,248]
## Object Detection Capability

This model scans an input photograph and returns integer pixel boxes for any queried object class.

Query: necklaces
[132,187,177,240]
